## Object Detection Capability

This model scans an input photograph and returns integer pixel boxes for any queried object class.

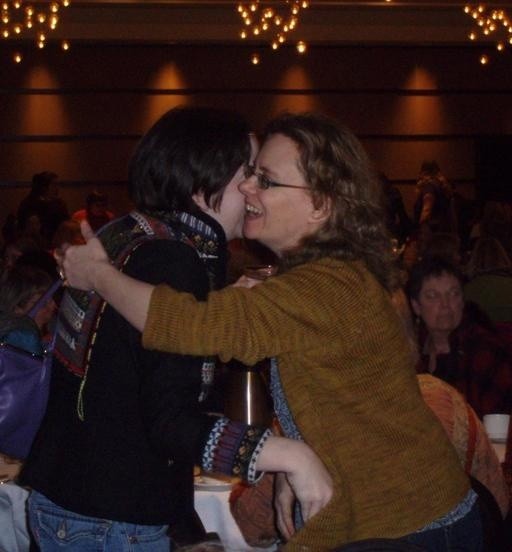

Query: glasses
[243,166,314,190]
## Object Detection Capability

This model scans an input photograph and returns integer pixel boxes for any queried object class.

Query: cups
[483,414,510,463]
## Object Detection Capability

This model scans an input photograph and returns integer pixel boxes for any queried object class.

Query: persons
[49,107,511,552]
[17,104,332,552]
[1,157,511,550]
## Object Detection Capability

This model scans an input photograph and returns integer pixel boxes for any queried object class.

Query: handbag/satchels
[0,309,56,460]
